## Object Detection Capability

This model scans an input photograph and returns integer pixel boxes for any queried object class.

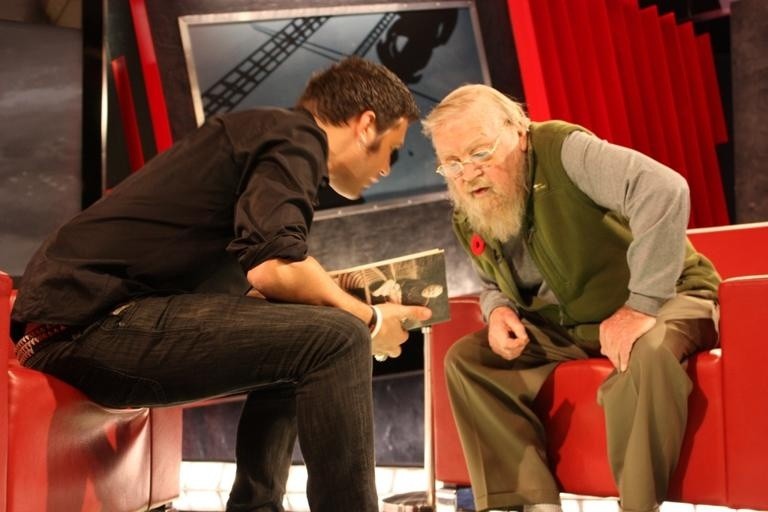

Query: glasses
[435,126,508,176]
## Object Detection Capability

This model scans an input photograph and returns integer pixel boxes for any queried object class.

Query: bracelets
[370,305,383,338]
[366,306,377,332]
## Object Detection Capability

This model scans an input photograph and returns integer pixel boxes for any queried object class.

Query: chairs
[430,218,767,509]
[0,266,181,511]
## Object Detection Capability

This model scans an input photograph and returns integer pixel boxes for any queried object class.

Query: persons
[422,83,721,512]
[399,279,443,307]
[12,58,431,512]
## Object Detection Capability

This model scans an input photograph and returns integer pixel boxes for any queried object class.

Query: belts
[13,321,68,365]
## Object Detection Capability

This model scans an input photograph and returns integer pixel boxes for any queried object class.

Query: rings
[374,356,386,362]
